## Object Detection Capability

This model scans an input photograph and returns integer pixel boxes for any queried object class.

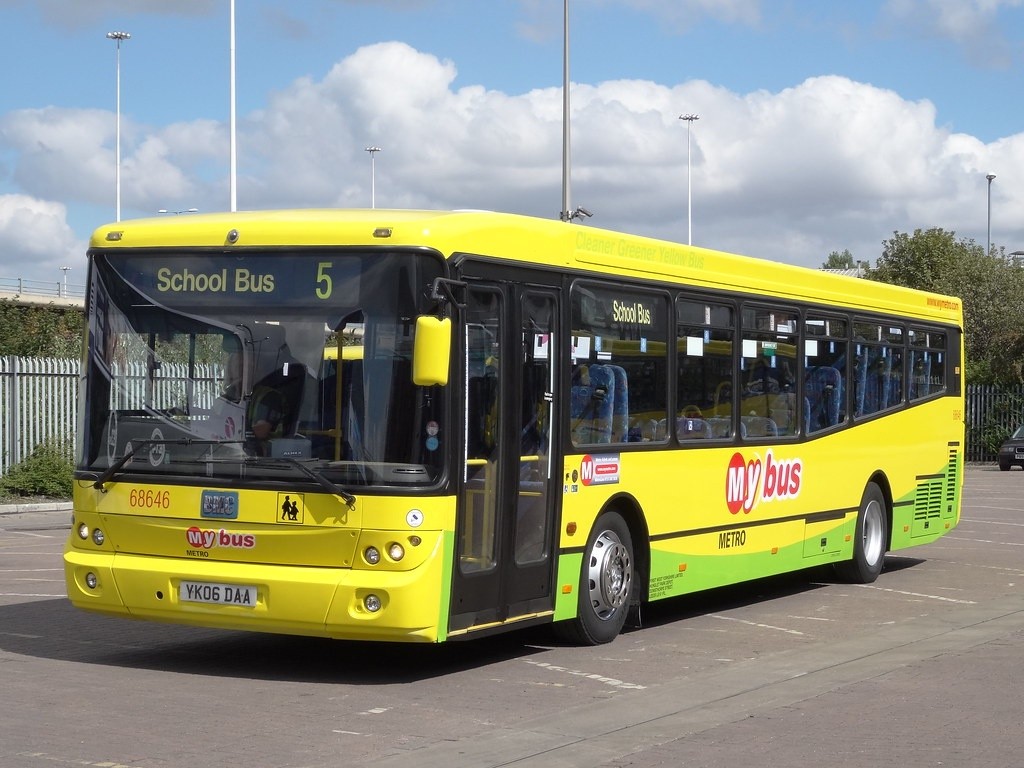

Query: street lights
[60,266,71,298]
[107,30,130,220]
[986,173,997,256]
[680,114,700,245]
[364,146,382,208]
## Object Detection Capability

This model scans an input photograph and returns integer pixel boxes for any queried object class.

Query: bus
[297,346,441,478]
[487,330,799,445]
[60,208,966,645]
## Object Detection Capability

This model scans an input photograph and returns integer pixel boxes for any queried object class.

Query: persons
[208,350,287,457]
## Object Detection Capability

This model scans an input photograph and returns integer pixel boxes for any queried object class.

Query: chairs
[225,335,932,492]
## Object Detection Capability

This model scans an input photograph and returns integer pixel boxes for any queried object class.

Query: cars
[996,425,1024,471]
